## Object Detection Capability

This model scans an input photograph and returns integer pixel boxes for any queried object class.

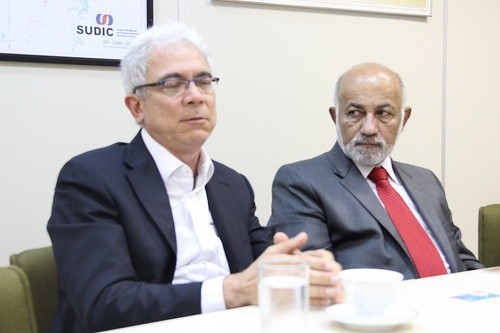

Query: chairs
[0,245,56,333]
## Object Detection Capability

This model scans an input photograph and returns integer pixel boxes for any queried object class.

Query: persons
[267,62,488,281]
[47,21,343,333]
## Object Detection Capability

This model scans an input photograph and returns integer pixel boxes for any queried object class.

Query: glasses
[133,76,221,97]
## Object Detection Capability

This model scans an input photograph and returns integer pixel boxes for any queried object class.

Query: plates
[323,301,417,331]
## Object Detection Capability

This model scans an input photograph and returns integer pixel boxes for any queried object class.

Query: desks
[94,267,500,333]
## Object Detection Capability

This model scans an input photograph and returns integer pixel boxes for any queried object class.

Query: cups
[332,268,403,300]
[255,256,310,333]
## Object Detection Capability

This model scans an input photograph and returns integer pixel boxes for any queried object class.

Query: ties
[367,166,449,277]
